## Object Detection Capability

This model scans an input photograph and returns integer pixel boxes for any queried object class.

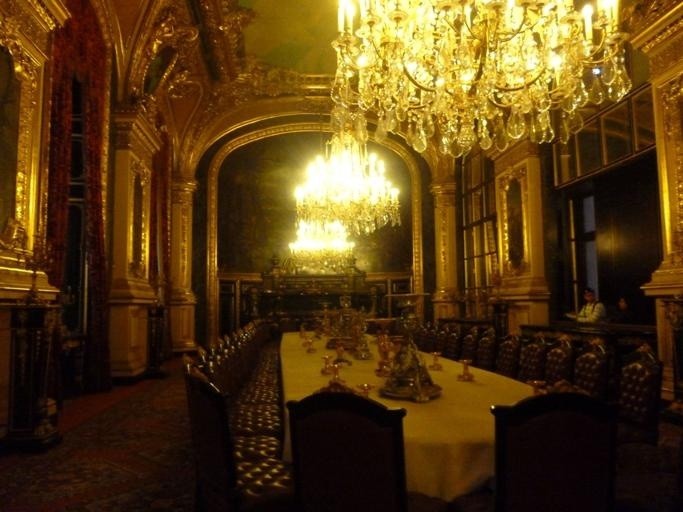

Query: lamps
[332,1,634,159]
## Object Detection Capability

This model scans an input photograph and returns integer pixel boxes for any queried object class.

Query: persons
[562,287,608,323]
[610,294,640,324]
